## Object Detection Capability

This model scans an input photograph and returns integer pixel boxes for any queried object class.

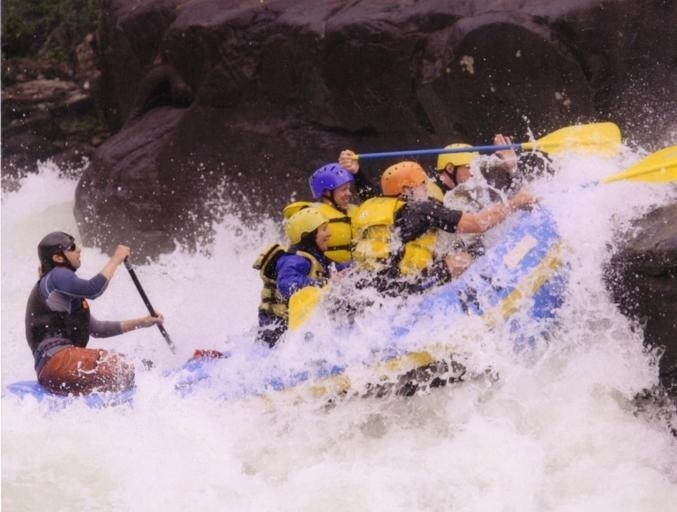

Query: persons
[285,134,544,297]
[24,229,166,397]
[252,208,354,347]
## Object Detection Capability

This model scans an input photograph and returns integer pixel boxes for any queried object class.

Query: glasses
[65,244,75,251]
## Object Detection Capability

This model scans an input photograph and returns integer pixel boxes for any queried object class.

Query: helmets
[286,207,329,245]
[308,162,354,200]
[436,143,481,171]
[381,161,428,196]
[38,232,74,260]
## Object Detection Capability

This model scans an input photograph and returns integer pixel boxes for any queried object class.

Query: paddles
[352,121,621,158]
[288,285,329,333]
[538,146,677,196]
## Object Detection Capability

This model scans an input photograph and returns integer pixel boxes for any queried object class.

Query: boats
[5,178,600,420]
